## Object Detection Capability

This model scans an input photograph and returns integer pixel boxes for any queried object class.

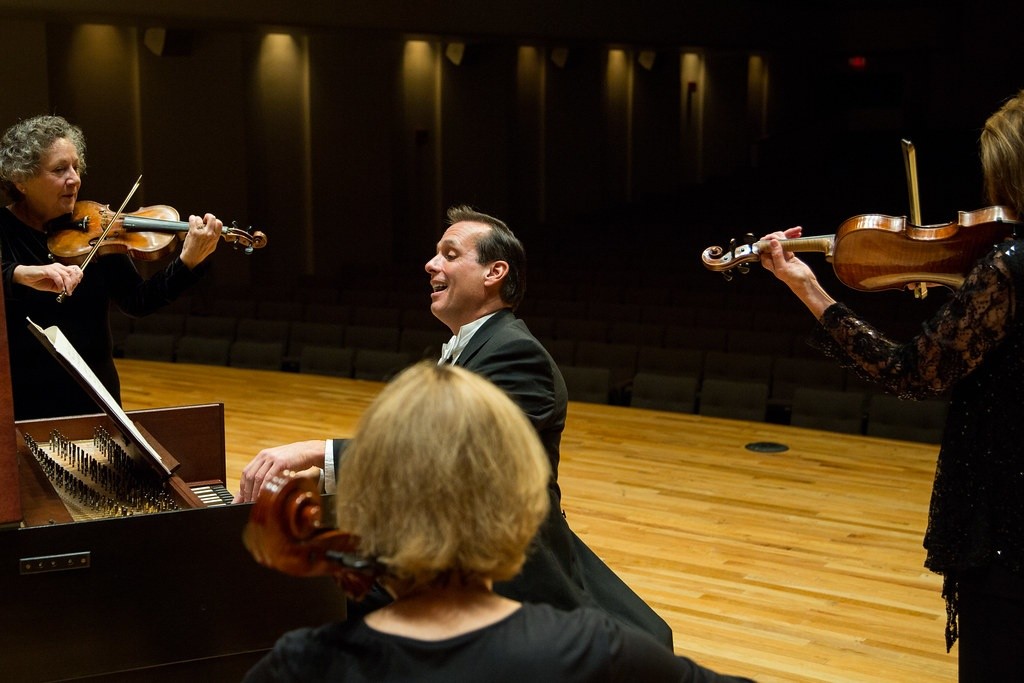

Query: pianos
[1,296,353,683]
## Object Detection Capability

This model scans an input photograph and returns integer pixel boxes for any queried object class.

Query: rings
[198,225,204,229]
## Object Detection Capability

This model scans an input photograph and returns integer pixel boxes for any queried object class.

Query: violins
[49,197,268,271]
[700,204,1019,291]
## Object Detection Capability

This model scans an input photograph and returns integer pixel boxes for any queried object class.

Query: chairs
[109,264,945,445]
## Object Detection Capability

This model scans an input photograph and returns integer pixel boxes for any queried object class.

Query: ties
[443,354,453,365]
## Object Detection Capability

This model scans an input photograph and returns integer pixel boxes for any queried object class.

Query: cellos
[243,467,395,593]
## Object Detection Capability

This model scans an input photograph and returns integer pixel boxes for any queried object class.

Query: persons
[243,359,756,683]
[760,95,1024,683]
[240,208,674,650]
[0,114,223,419]
